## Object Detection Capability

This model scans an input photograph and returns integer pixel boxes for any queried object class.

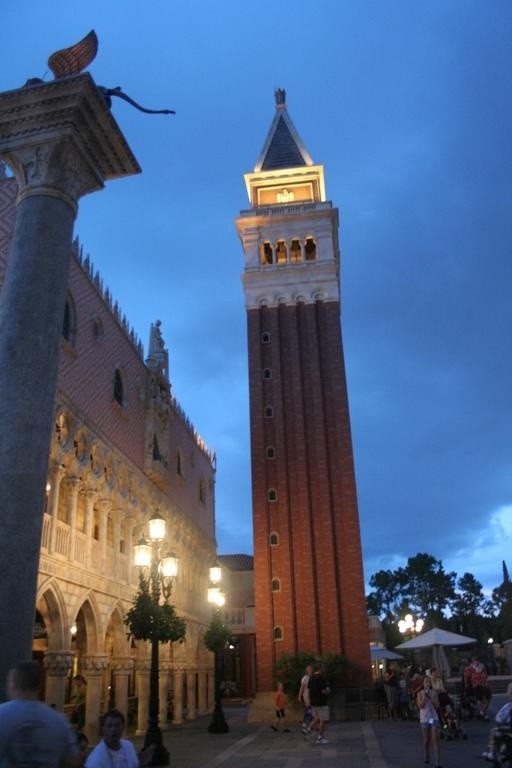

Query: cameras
[425,689,432,694]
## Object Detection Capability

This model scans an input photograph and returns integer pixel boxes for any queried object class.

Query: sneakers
[269,721,292,733]
[302,726,329,744]
[475,711,490,722]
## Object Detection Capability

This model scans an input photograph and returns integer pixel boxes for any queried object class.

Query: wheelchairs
[482,708,512,768]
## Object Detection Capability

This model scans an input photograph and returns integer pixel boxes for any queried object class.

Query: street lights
[397,613,425,669]
[206,558,231,734]
[132,506,180,768]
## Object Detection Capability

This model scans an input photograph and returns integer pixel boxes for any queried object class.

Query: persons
[297,665,321,732]
[373,654,511,768]
[269,681,292,733]
[300,662,332,745]
[71,674,90,748]
[82,707,140,768]
[0,660,83,767]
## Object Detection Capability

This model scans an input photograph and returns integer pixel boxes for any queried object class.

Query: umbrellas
[393,625,479,651]
[371,644,404,681]
[430,646,452,679]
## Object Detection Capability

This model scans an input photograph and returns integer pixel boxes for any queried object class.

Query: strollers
[435,691,468,740]
